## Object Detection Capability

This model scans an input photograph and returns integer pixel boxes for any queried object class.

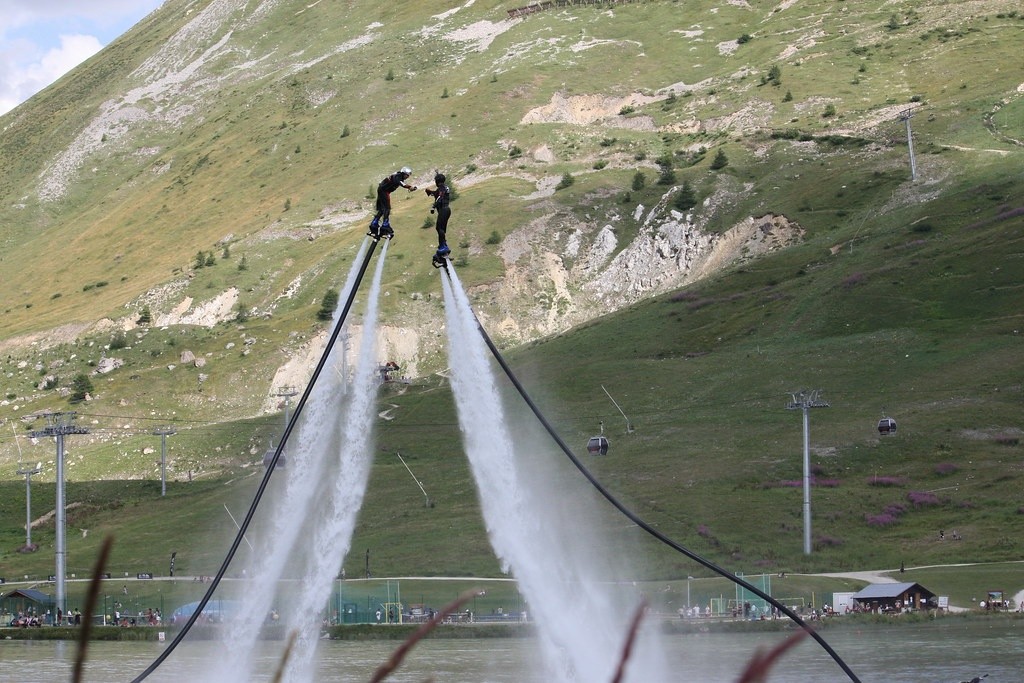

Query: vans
[171,599,254,626]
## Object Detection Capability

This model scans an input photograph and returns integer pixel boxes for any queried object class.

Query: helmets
[435,174,445,187]
[401,166,411,176]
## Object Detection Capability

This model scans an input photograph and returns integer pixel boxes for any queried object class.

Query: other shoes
[370,218,378,234]
[438,242,451,256]
[381,219,394,233]
[433,254,441,260]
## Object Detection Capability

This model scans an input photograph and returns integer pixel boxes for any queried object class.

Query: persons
[369,166,417,234]
[425,174,451,253]
[1,598,1024,630]
